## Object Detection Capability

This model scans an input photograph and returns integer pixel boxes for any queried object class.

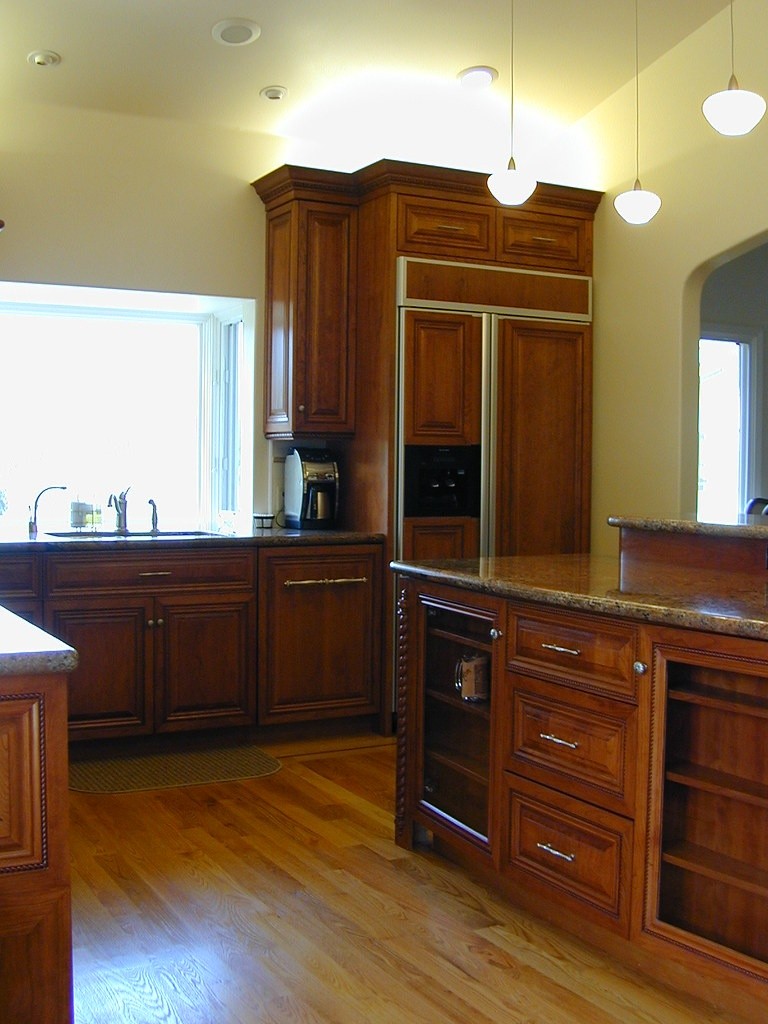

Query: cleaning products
[70,491,102,532]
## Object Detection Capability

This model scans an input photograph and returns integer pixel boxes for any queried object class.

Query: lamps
[613,0,662,225]
[702,0,766,136]
[487,0,538,206]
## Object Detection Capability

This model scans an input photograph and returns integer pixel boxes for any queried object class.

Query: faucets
[107,485,135,532]
[28,487,67,533]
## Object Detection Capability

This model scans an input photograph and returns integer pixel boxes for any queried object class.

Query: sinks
[45,530,223,537]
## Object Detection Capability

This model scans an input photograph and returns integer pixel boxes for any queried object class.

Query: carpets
[68,732,283,793]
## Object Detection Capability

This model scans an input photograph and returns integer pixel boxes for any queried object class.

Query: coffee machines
[282,444,341,530]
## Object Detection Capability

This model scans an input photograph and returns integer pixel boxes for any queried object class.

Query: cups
[454,645,489,703]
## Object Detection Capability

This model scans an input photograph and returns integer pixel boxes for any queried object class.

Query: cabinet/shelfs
[258,544,383,731]
[494,317,592,557]
[0,551,43,628]
[393,572,768,1024]
[44,547,257,739]
[401,516,479,561]
[264,200,358,433]
[0,672,75,1024]
[396,192,594,278]
[403,310,481,448]
